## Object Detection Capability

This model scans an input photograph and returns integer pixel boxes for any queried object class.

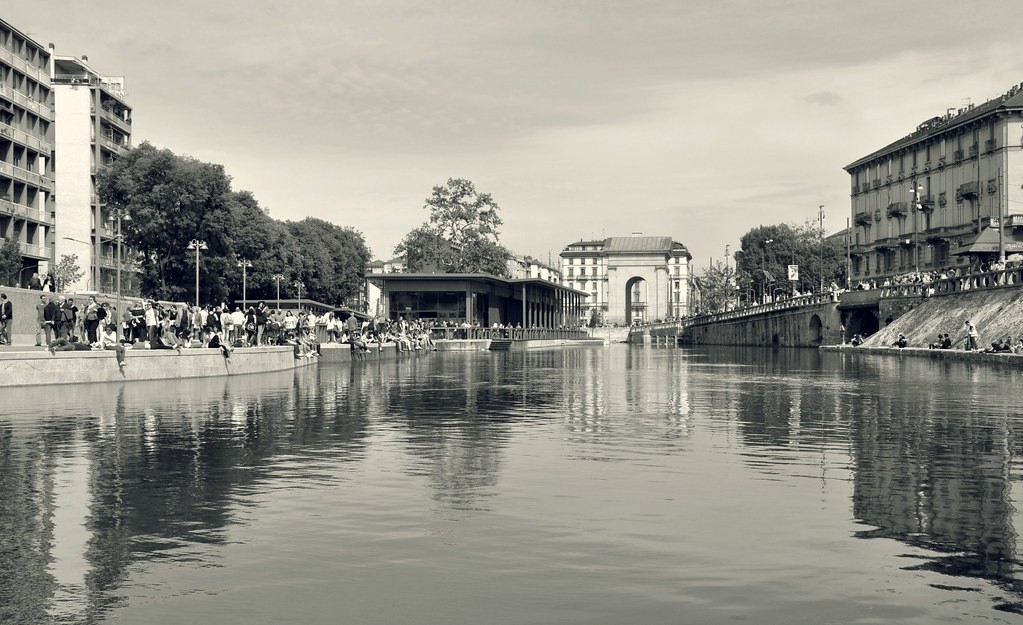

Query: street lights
[187,238,209,309]
[107,207,133,344]
[765,231,773,302]
[293,281,305,318]
[818,205,826,293]
[271,272,285,312]
[236,257,253,313]
[63,234,123,292]
[910,172,923,278]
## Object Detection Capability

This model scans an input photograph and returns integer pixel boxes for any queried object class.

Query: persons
[752,253,1019,307]
[851,331,864,347]
[838,324,847,346]
[964,320,979,350]
[978,336,1023,354]
[892,334,906,348]
[0,294,13,346]
[885,315,893,326]
[35,293,538,367]
[929,334,951,348]
